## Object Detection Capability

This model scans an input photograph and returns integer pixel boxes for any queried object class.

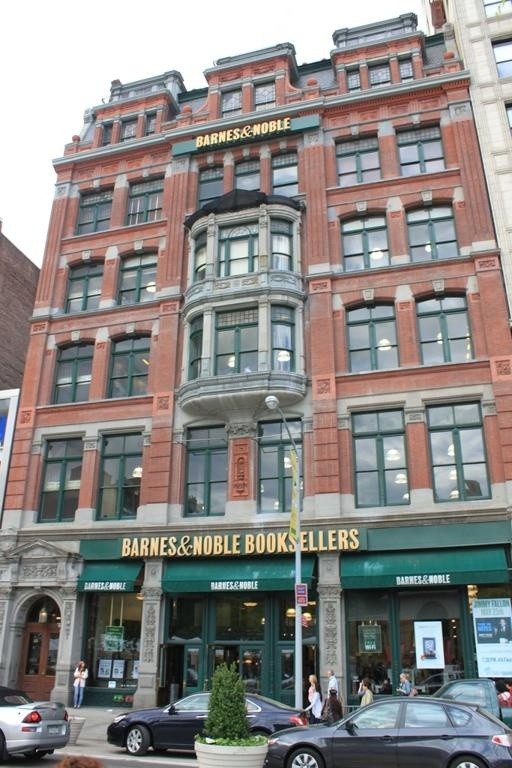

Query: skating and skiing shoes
[73,704,81,709]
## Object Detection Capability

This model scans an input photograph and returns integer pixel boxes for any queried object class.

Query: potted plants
[195,660,268,768]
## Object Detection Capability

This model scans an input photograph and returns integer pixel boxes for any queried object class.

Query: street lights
[255,390,307,712]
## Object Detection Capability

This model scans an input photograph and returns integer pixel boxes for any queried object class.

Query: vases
[68,716,87,746]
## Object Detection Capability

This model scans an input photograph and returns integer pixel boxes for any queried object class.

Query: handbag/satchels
[407,688,418,697]
[322,706,334,725]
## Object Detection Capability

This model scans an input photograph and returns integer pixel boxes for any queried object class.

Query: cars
[181,666,300,692]
[262,696,512,768]
[0,684,73,766]
[103,691,314,758]
[385,664,464,695]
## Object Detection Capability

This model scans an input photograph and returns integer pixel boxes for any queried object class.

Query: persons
[497,619,512,643]
[498,678,512,707]
[296,669,374,724]
[72,660,89,708]
[396,672,411,696]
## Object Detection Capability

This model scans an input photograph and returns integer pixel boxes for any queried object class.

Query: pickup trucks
[432,678,512,728]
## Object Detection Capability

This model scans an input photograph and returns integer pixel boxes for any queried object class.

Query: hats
[329,689,338,695]
